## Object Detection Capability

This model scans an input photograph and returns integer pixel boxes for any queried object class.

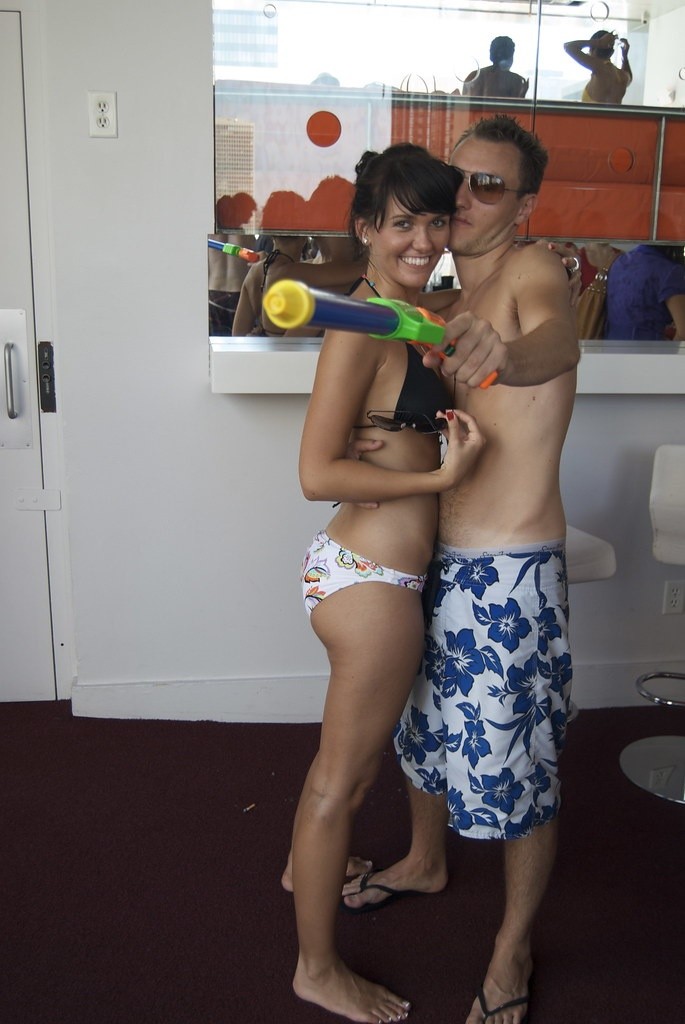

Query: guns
[207,238,262,265]
[262,277,499,391]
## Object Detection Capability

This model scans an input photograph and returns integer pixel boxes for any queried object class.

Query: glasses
[367,408,454,435]
[447,165,532,206]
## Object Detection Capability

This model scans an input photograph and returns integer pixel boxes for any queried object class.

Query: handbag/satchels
[573,252,619,340]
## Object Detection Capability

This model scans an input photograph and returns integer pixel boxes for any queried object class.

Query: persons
[279,115,582,1023]
[538,239,685,342]
[564,31,633,105]
[208,174,369,338]
[462,36,529,97]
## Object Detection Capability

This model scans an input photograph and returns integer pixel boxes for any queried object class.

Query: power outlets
[87,91,118,138]
[662,580,685,615]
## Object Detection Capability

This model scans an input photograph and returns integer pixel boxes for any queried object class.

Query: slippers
[472,954,536,1024]
[340,867,448,914]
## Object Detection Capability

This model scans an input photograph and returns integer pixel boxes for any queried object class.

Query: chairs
[620,443,684,804]
[566,525,616,727]
